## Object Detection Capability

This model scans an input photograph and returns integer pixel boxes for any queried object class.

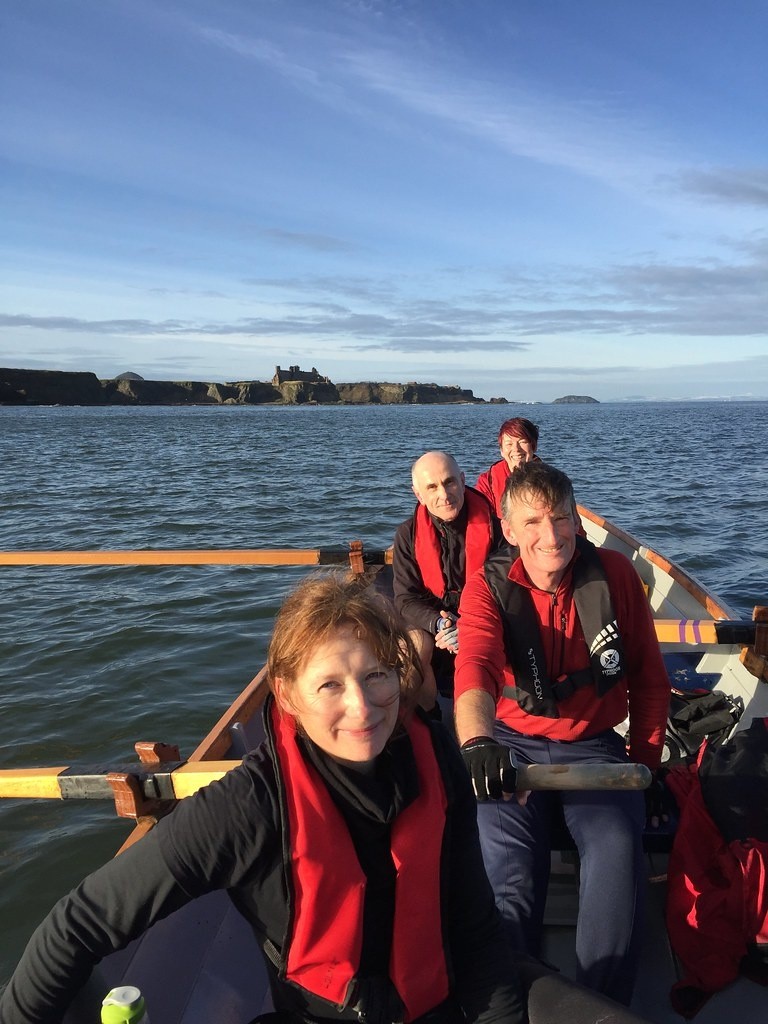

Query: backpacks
[663,688,743,757]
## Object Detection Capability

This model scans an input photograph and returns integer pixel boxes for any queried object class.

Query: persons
[475,418,541,516]
[0,578,653,1024]
[393,452,502,721]
[454,462,672,1000]
[668,716,768,1024]
